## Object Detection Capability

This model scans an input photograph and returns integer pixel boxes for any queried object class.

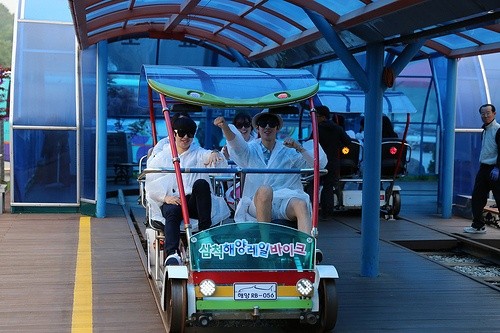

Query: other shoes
[165,255,182,265]
[321,215,330,221]
[306,248,323,264]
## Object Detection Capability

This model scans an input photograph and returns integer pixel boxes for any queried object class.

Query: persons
[309,105,358,222]
[145,117,229,266]
[463,103,500,233]
[147,111,201,166]
[214,109,328,269]
[221,111,256,218]
[330,115,399,189]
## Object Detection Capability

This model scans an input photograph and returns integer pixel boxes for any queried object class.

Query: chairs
[381,138,412,179]
[147,147,312,230]
[338,138,364,179]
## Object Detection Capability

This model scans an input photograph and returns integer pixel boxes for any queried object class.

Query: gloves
[490,167,500,180]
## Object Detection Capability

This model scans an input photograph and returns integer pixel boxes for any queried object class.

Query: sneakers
[464,226,486,233]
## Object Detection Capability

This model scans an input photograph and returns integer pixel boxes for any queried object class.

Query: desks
[114,163,146,184]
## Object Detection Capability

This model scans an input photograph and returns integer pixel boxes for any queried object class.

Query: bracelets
[212,150,219,153]
[296,146,302,153]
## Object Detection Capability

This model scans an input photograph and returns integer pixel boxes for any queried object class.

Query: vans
[405,135,437,174]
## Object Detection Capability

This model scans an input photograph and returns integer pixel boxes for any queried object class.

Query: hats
[251,108,283,132]
[173,118,196,130]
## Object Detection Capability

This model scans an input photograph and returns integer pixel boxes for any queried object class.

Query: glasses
[257,122,278,128]
[235,121,250,129]
[176,129,195,138]
